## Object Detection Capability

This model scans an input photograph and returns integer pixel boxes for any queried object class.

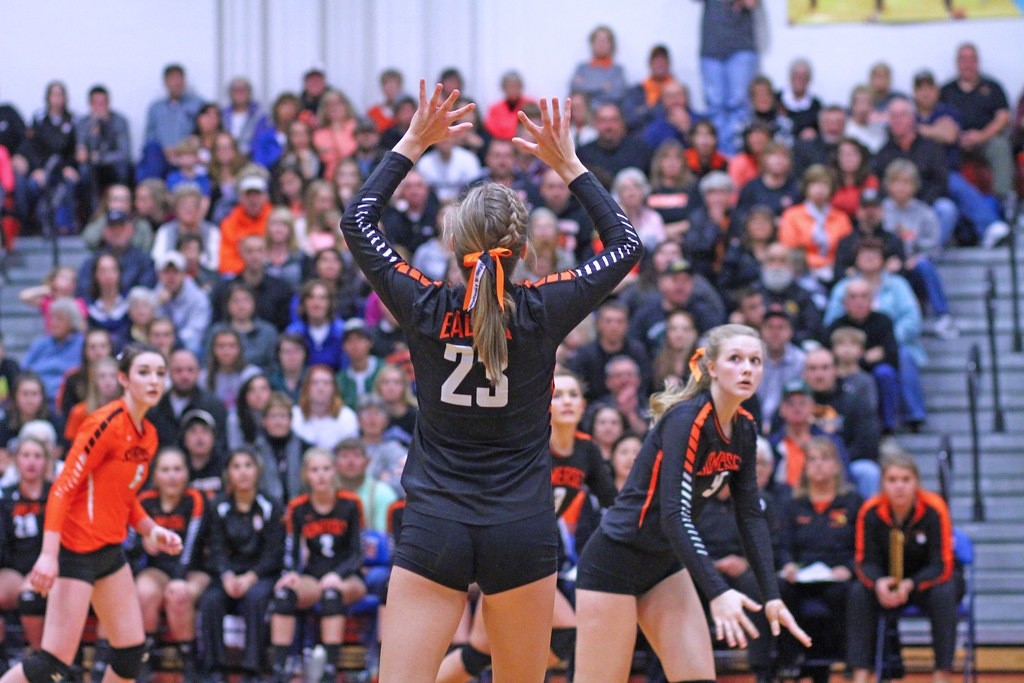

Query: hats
[659,257,693,275]
[338,318,374,345]
[698,171,735,197]
[238,175,268,192]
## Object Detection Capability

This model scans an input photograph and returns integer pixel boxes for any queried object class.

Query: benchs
[0,237,1024,643]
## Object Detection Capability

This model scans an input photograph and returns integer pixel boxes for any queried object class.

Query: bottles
[310,645,326,682]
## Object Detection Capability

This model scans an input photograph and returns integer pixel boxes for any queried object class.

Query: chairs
[870,529,977,683]
[288,528,394,683]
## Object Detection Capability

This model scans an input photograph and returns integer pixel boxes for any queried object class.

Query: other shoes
[73,633,381,682]
[980,220,1010,247]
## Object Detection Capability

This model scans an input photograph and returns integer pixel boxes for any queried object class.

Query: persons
[0,0,1024,683]
[340,78,646,683]
